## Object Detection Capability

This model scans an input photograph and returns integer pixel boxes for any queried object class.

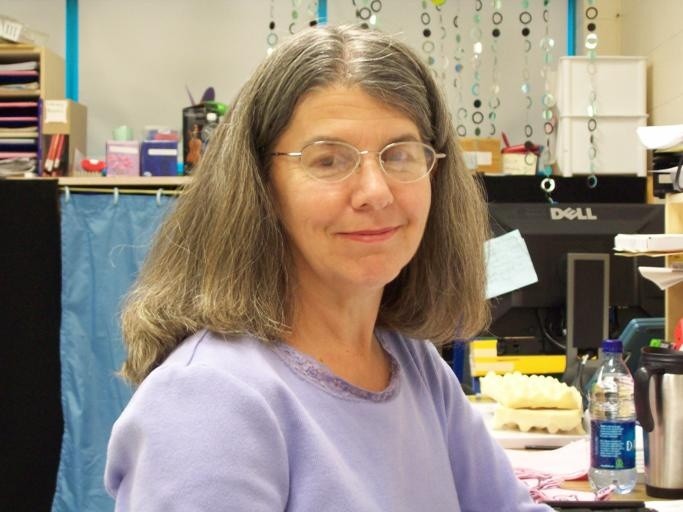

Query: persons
[101,23,556,512]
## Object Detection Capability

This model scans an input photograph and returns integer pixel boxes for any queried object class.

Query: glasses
[266,140,446,184]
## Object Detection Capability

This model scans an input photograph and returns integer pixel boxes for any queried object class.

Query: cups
[631,343,682,500]
[181,104,219,175]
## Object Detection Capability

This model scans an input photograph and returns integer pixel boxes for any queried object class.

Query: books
[0,59,38,177]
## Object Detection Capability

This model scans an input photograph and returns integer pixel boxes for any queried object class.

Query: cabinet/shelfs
[554,56,648,176]
[0,44,65,177]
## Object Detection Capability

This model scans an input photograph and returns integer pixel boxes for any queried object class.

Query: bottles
[589,339,639,496]
